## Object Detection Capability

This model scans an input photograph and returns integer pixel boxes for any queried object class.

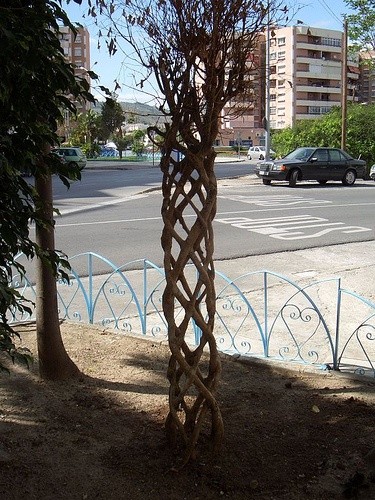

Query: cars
[49,148,86,171]
[248,146,276,160]
[254,147,367,188]
